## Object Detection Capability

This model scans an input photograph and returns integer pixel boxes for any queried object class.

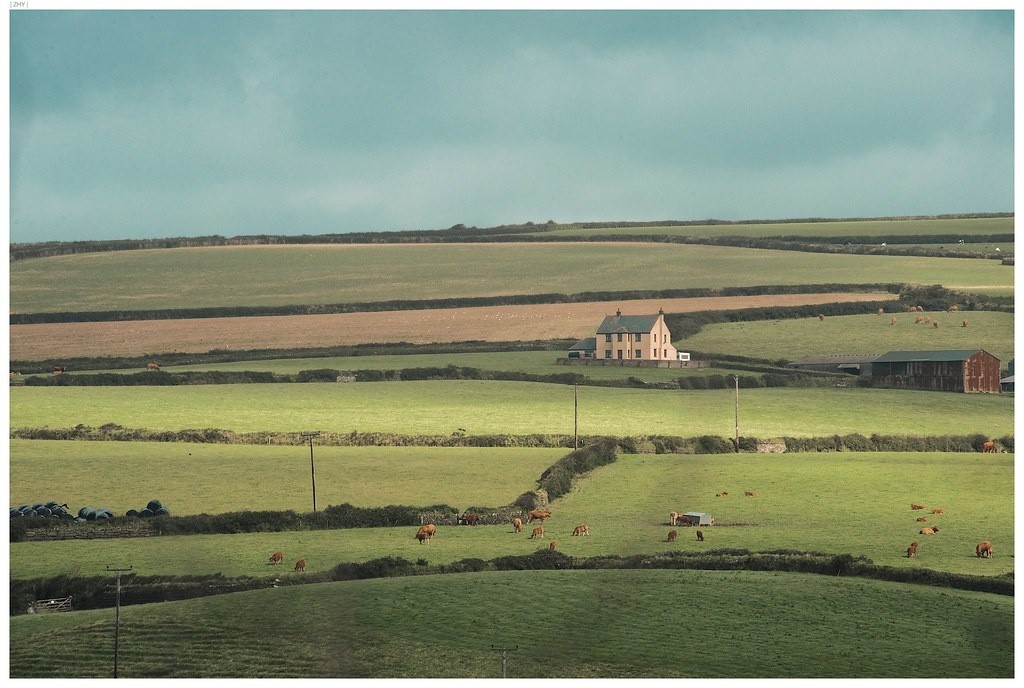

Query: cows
[550,541,557,552]
[745,492,756,496]
[696,530,704,541]
[526,510,551,524]
[669,512,694,527]
[295,560,305,572]
[414,524,437,544]
[983,441,996,453]
[668,530,677,542]
[906,503,945,559]
[975,540,993,559]
[717,492,728,497]
[531,527,544,539]
[270,552,284,565]
[572,524,590,536]
[514,518,522,534]
[818,305,969,328]
